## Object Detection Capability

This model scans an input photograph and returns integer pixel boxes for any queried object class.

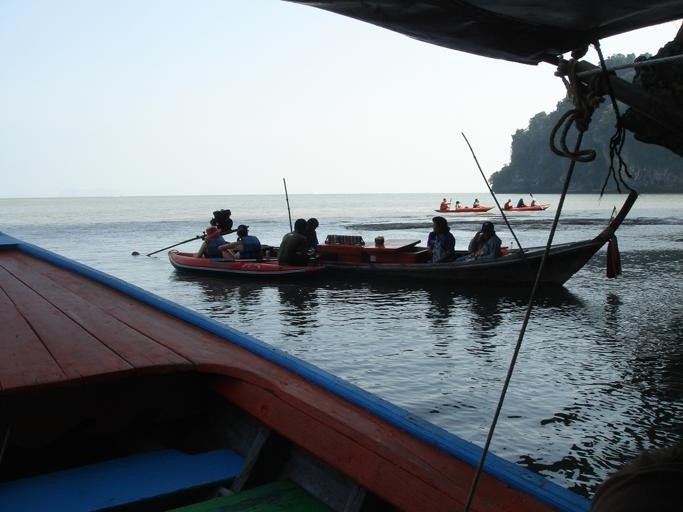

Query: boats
[2,226,592,511]
[317,188,640,291]
[167,245,328,280]
[433,204,495,213]
[500,202,551,211]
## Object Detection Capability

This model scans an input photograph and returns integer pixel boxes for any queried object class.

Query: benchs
[0,447,247,512]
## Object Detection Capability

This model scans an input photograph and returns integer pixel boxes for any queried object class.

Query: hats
[204,225,221,240]
[237,225,249,235]
[481,222,494,235]
[308,218,318,229]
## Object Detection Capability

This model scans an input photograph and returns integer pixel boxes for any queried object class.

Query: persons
[455,201,468,210]
[516,198,527,208]
[305,218,319,249]
[425,216,456,263]
[216,223,261,260]
[439,196,452,210]
[452,222,503,263]
[503,199,513,209]
[530,199,540,207]
[193,227,228,259]
[473,199,481,208]
[277,218,320,267]
